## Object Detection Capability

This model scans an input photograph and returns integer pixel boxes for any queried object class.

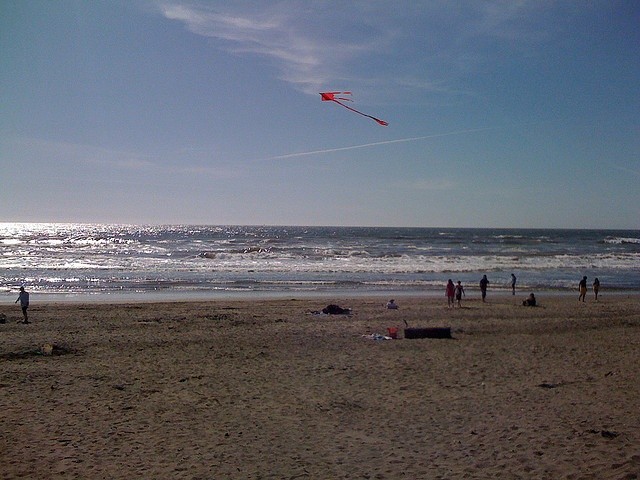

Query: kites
[320,91,390,126]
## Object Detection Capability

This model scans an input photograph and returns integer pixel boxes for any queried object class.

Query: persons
[15,287,29,324]
[446,279,455,307]
[479,275,490,303]
[578,275,588,302]
[387,298,399,308]
[522,293,537,307]
[457,280,466,306]
[592,277,601,300]
[511,273,518,295]
